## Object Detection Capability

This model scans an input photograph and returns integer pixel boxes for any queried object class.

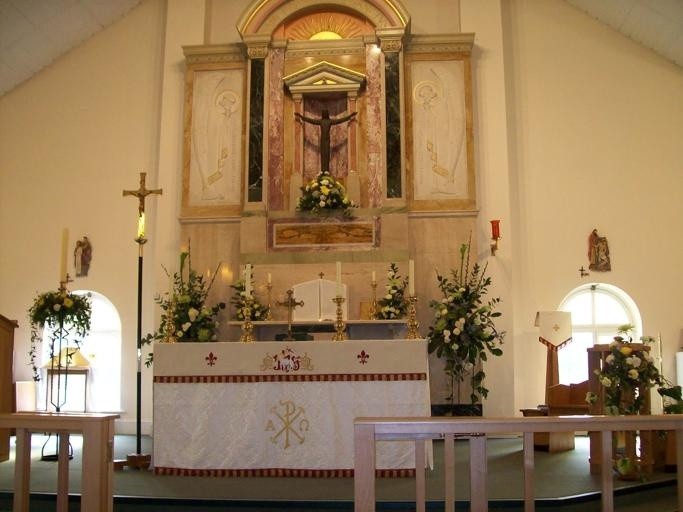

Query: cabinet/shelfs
[150,340,433,477]
[519,379,635,454]
[0,313,20,463]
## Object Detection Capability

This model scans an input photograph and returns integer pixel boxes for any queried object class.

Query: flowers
[428,230,506,417]
[142,252,222,367]
[230,274,267,322]
[585,323,683,415]
[372,262,408,319]
[295,170,356,215]
[28,291,92,382]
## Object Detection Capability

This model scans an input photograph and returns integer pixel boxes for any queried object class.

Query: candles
[267,272,272,285]
[372,271,375,281]
[336,262,341,297]
[408,259,415,296]
[245,263,252,297]
